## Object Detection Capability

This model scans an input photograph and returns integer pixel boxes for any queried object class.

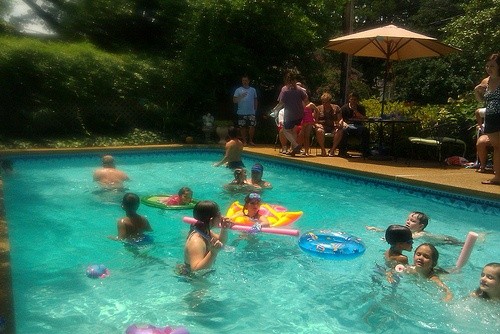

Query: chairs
[270,110,361,157]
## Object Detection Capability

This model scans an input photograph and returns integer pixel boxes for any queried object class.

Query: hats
[251,164,263,174]
[244,192,262,203]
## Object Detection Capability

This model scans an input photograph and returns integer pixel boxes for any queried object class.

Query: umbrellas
[323,21,462,146]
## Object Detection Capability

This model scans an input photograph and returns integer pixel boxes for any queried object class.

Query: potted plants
[215,120,233,144]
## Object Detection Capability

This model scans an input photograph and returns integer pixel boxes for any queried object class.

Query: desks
[349,119,420,161]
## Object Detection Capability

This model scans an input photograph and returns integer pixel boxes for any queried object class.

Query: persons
[473,54,500,186]
[93,69,365,275]
[367,211,500,302]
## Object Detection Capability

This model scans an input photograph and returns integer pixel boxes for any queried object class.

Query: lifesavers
[395,231,479,273]
[142,194,200,210]
[182,216,299,236]
[300,231,366,258]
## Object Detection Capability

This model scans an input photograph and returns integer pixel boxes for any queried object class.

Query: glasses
[248,193,260,199]
[393,237,414,244]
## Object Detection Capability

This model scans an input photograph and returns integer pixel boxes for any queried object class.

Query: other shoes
[321,150,335,157]
[286,150,301,155]
[481,179,500,184]
[475,163,488,173]
[290,145,302,156]
